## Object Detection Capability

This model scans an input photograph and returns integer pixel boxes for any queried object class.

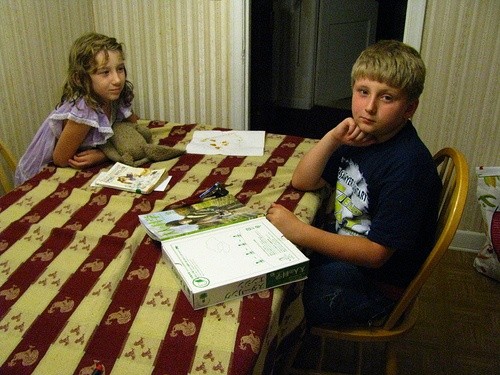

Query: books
[137,193,310,310]
[91,162,173,195]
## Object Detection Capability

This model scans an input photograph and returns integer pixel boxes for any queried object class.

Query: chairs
[0,140,19,197]
[278,146,469,375]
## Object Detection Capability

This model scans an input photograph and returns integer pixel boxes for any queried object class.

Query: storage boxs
[160,216,310,311]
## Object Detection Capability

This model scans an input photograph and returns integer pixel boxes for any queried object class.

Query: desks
[0,120,329,375]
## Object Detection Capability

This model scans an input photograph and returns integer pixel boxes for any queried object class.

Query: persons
[265,40,445,330]
[13,33,135,189]
